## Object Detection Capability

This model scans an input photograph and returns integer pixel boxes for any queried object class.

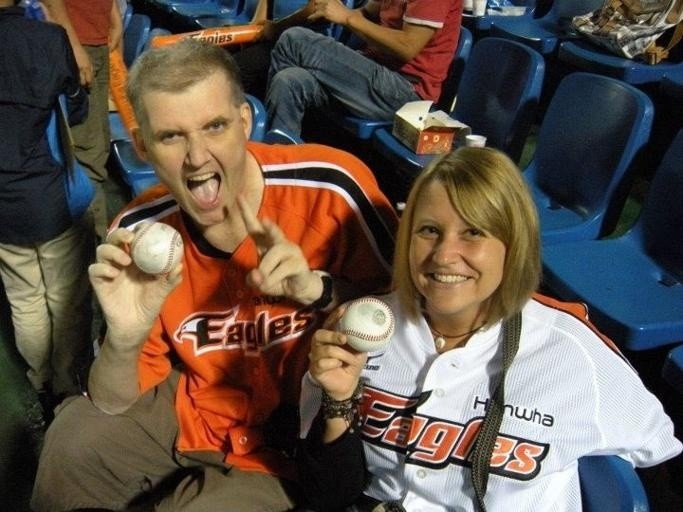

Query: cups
[465,134,487,147]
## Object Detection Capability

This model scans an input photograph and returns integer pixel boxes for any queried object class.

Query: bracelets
[319,381,368,433]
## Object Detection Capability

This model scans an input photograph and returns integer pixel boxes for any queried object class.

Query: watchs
[304,268,333,314]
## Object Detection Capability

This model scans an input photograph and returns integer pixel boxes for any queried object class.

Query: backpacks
[566,0,683,67]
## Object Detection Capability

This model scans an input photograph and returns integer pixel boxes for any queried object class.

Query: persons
[221,1,337,95]
[298,145,683,511]
[262,1,465,144]
[0,0,99,429]
[30,36,401,512]
[37,0,122,247]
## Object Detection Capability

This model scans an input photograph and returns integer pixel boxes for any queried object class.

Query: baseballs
[341,297,395,353]
[130,223,184,275]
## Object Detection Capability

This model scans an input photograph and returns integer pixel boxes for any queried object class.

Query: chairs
[107,1,682,512]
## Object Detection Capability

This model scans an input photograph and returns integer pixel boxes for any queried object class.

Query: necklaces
[422,312,487,349]
